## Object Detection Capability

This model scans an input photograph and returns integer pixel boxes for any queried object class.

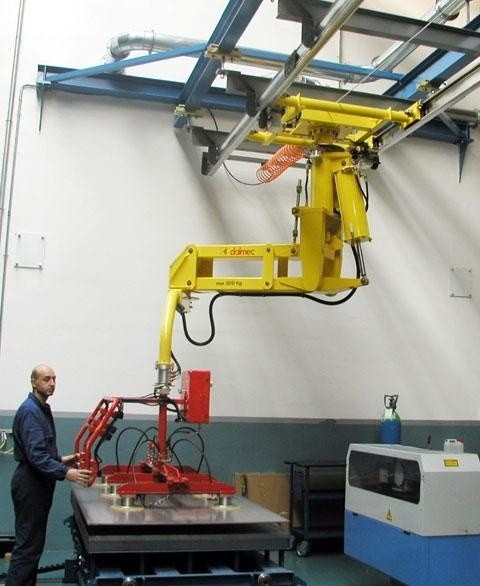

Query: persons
[5,365,92,585]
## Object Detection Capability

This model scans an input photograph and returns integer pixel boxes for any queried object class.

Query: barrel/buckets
[444,439,464,452]
